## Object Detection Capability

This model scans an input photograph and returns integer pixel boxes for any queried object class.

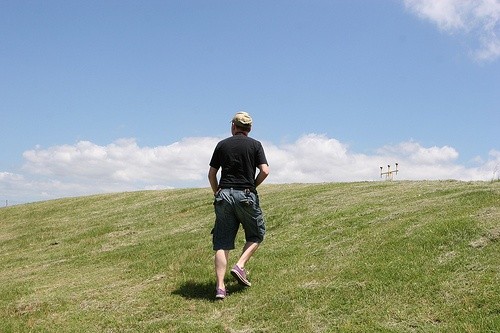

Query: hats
[228,112,253,128]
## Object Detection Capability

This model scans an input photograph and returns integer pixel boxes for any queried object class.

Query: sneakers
[231,265,252,287]
[215,288,230,299]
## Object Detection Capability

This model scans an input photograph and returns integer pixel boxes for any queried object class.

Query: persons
[208,111,270,299]
